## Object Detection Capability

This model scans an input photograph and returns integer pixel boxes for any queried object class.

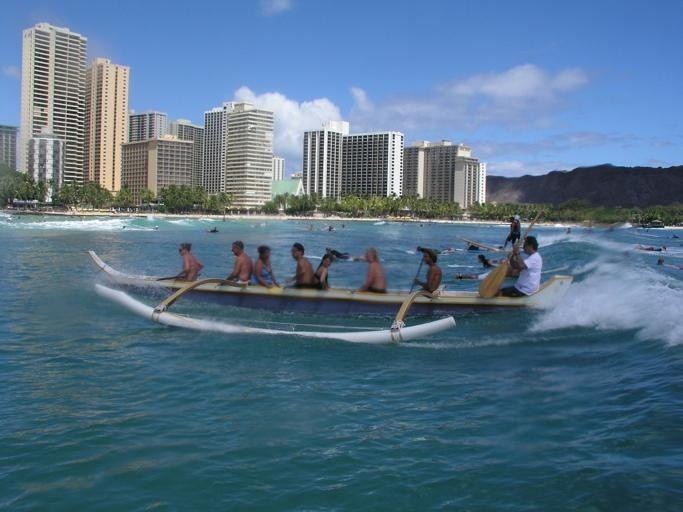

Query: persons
[509,217,519,248]
[221,240,253,286]
[326,247,349,260]
[250,245,282,289]
[478,255,494,268]
[494,236,543,298]
[286,242,314,289]
[313,253,333,290]
[504,215,520,248]
[363,247,387,294]
[634,244,667,251]
[448,247,456,253]
[671,234,680,239]
[175,243,204,283]
[414,248,442,298]
[657,258,683,271]
[454,259,505,280]
[566,228,571,234]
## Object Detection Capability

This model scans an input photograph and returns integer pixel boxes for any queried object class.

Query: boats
[87,248,574,345]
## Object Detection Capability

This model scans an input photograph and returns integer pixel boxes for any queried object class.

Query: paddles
[479,209,543,298]
